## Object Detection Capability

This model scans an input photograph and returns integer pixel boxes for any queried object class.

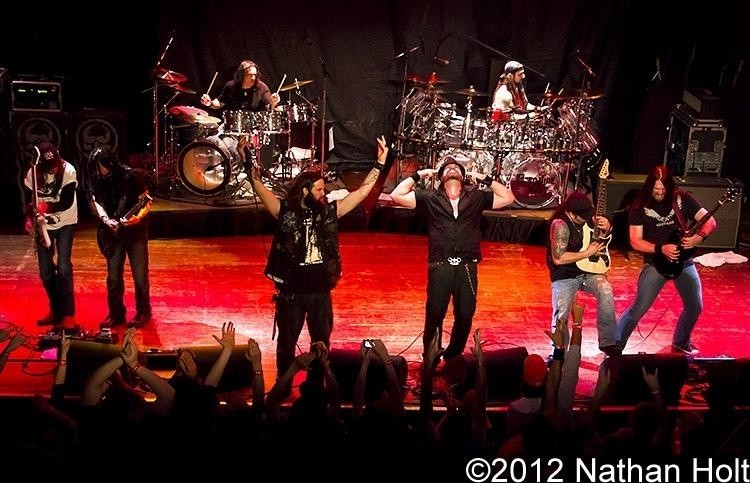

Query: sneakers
[265,377,296,405]
[600,344,620,357]
[672,342,703,354]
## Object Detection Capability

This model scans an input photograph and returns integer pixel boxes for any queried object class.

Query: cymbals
[276,105,291,111]
[478,106,494,111]
[152,67,188,83]
[416,86,453,94]
[182,115,221,123]
[169,106,207,117]
[394,71,451,84]
[586,93,604,98]
[160,81,197,95]
[527,89,566,100]
[453,89,490,96]
[279,79,314,92]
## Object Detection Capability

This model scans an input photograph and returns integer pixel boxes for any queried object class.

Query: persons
[618,166,716,355]
[492,59,549,120]
[87,145,153,332]
[546,191,622,359]
[389,156,516,368]
[24,142,78,327]
[236,134,388,382]
[21,322,265,482]
[201,60,281,117]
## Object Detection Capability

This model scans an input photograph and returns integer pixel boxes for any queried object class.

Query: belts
[432,256,475,266]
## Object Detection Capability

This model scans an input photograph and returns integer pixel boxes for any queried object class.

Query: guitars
[29,144,51,248]
[653,177,746,280]
[96,191,154,260]
[576,158,610,275]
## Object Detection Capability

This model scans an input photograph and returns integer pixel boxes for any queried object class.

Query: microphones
[236,134,252,156]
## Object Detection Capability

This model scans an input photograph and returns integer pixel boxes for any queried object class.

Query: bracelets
[654,243,662,257]
[696,232,708,242]
[483,176,492,184]
[207,101,214,109]
[100,215,107,218]
[411,173,420,182]
[607,225,612,233]
[374,161,385,173]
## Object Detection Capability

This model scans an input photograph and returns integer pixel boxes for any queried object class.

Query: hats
[499,60,523,78]
[565,191,596,220]
[438,157,467,176]
[41,143,59,164]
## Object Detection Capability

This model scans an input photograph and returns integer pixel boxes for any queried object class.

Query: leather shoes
[99,315,125,331]
[127,312,152,328]
[37,313,65,326]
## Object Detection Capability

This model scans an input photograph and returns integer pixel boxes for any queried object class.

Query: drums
[283,103,311,124]
[255,109,288,132]
[443,116,488,150]
[223,109,255,131]
[491,111,557,151]
[433,140,495,191]
[400,89,456,145]
[177,132,244,196]
[500,151,561,209]
[558,99,602,171]
